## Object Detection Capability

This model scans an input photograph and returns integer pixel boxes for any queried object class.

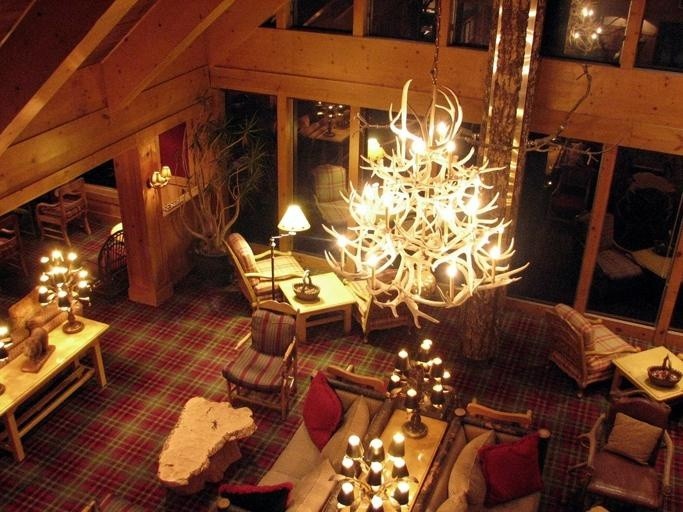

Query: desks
[0,316,116,464]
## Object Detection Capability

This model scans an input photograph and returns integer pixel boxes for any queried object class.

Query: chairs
[0,207,28,285]
[30,176,95,252]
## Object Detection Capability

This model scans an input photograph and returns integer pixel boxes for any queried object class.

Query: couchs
[0,284,83,369]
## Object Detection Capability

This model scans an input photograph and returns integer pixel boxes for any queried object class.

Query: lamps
[318,3,532,326]
[144,162,174,194]
[29,240,101,338]
